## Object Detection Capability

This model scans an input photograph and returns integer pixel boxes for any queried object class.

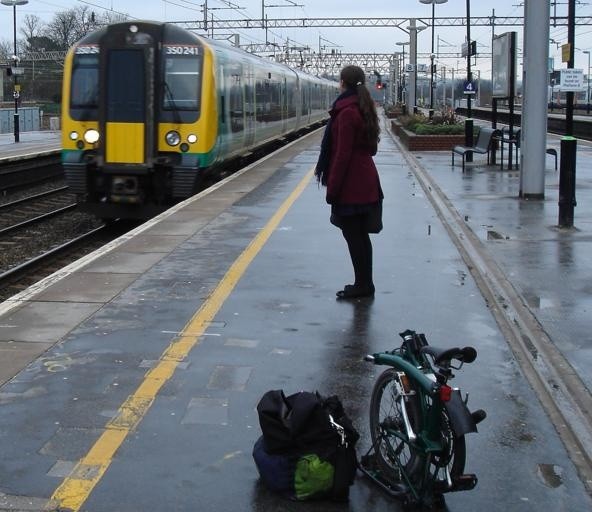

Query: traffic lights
[377,83,388,90]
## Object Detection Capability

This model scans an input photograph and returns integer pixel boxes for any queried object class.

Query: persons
[314,65,384,298]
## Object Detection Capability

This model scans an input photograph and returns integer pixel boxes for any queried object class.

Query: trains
[57,17,344,213]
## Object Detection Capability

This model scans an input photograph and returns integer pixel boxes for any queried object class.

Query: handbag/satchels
[252,389,359,503]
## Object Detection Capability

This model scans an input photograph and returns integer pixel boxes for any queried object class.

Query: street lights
[2,1,31,142]
[388,24,428,118]
[582,50,591,108]
[419,0,449,118]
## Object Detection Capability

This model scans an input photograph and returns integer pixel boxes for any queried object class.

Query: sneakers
[337,285,374,298]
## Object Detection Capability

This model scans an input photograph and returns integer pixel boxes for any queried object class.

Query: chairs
[502,126,557,171]
[452,127,500,172]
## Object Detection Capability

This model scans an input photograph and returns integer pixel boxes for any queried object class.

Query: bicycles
[358,320,485,512]
